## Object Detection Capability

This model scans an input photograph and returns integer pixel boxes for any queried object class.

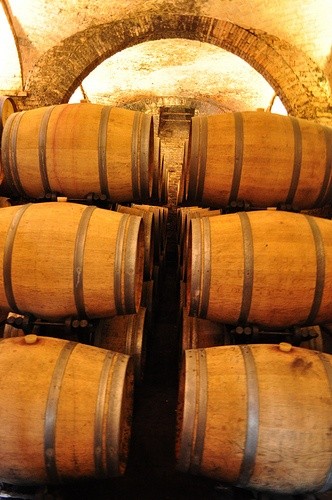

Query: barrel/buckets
[1,95,332,494]
